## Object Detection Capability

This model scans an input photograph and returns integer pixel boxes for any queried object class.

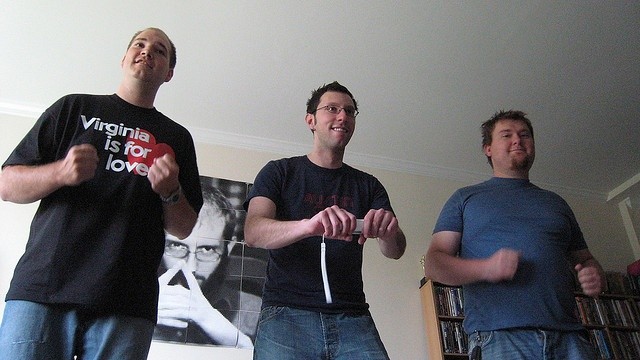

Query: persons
[149,182,262,348]
[242,80,406,360]
[425,107,606,360]
[0,27,204,360]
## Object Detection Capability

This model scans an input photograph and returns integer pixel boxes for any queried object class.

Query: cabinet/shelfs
[421,271,640,360]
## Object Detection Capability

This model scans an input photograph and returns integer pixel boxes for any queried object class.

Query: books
[439,320,469,354]
[575,296,603,325]
[587,328,615,359]
[600,299,640,326]
[614,330,640,360]
[569,268,584,293]
[432,284,465,318]
[605,271,640,294]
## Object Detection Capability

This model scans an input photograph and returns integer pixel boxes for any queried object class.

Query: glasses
[317,105,359,118]
[164,246,223,262]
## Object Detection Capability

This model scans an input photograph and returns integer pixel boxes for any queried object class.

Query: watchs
[158,180,183,204]
